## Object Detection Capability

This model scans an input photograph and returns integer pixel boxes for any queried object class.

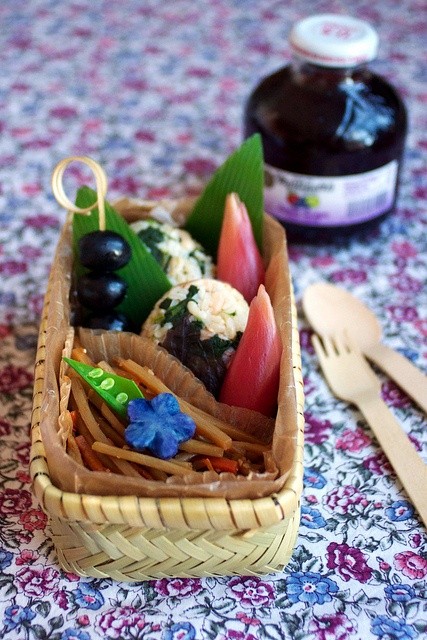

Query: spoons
[302,281,427,414]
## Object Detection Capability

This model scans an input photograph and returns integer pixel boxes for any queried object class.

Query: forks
[310,332,427,559]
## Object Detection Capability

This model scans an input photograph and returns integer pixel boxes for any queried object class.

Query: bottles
[245,10,416,248]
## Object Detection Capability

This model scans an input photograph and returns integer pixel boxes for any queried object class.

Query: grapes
[70,312,126,333]
[76,271,130,312]
[77,230,132,270]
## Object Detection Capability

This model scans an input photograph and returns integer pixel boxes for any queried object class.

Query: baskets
[30,194,304,582]
